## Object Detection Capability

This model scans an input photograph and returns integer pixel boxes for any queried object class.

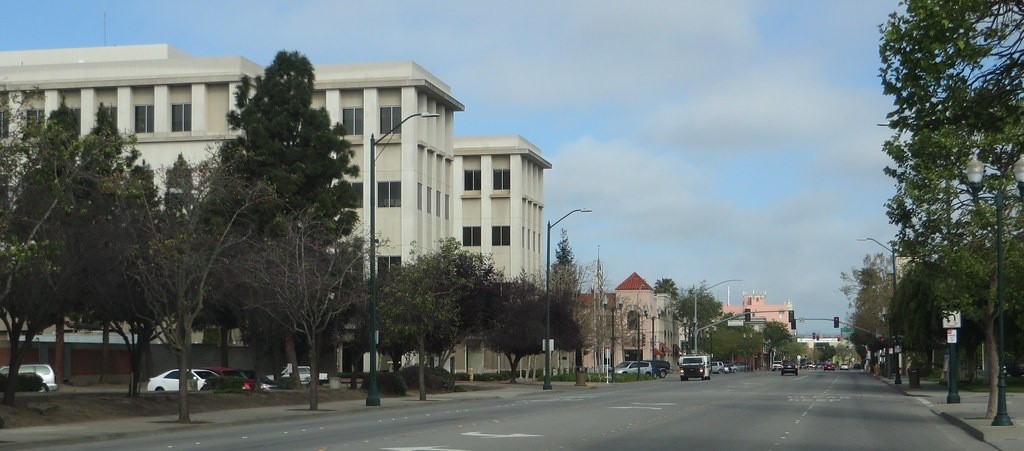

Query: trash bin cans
[907,367,920,387]
[575,366,587,386]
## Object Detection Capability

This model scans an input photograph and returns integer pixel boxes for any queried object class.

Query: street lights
[857,236,902,385]
[365,111,439,406]
[967,155,1024,425]
[544,208,592,391]
[694,279,743,353]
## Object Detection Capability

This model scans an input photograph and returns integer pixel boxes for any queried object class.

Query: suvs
[648,360,670,378]
[0,364,57,393]
[613,360,652,380]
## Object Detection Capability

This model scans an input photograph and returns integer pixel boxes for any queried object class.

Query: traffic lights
[745,308,750,322]
[789,311,797,329]
[833,317,840,329]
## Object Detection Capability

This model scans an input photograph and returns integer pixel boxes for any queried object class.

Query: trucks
[678,355,711,381]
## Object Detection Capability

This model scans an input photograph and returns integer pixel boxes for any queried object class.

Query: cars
[265,364,329,387]
[772,359,850,375]
[711,361,748,373]
[147,369,220,391]
[146,364,329,392]
